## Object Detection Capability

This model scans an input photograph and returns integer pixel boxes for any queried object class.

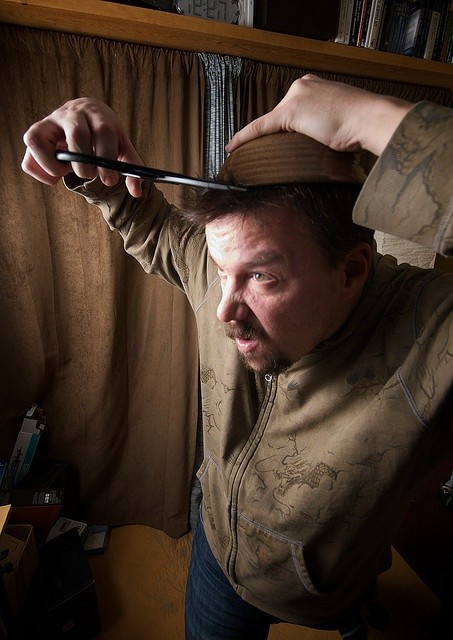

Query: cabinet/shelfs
[0,0,453,91]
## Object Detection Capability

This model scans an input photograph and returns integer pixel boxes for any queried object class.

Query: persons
[19,73,453,640]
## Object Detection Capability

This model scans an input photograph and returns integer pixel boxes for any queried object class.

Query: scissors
[56,152,245,190]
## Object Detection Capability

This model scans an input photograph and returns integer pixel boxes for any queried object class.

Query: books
[338,0,353,45]
[350,0,366,48]
[404,0,440,61]
[432,0,453,64]
[380,0,410,55]
[361,0,386,52]
